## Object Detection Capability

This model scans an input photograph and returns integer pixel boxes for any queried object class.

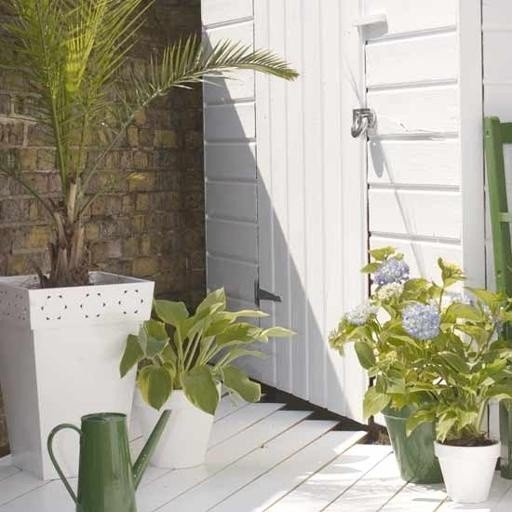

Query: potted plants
[0,0,304,485]
[421,287,512,505]
[118,287,297,476]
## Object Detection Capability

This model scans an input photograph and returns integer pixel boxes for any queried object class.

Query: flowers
[323,244,512,445]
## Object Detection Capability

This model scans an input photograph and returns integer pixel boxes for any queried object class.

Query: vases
[377,395,448,486]
